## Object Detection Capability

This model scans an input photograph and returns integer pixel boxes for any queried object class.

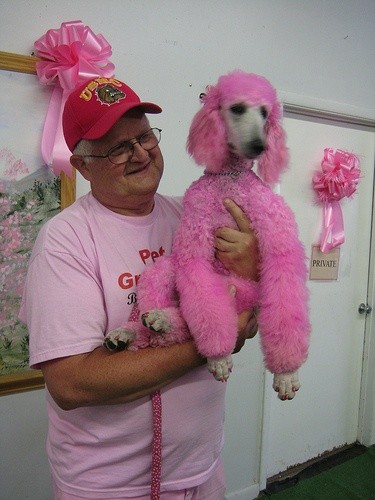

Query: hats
[62,77,163,153]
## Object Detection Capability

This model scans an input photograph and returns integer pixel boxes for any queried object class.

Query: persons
[21,77,262,500]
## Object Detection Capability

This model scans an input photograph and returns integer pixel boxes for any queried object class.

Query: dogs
[102,71,312,401]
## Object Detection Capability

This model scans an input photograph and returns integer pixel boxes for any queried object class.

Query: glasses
[81,127,161,165]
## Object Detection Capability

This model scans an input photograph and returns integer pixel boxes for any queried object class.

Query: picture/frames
[0,49,76,396]
